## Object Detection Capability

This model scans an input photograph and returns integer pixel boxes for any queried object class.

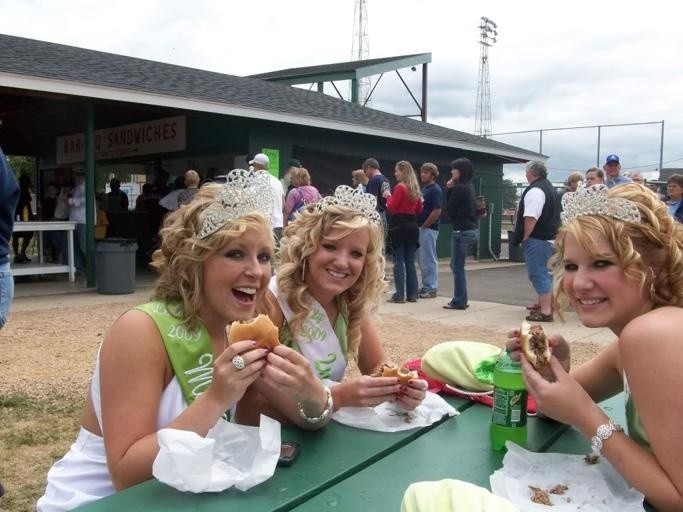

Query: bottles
[16,215,20,222]
[22,206,29,222]
[490,347,528,453]
[477,196,487,217]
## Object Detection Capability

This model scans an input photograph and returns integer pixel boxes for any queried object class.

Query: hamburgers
[520,318,551,367]
[370,360,419,398]
[224,312,284,377]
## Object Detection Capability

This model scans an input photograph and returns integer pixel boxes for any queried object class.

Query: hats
[605,154,620,165]
[248,154,268,165]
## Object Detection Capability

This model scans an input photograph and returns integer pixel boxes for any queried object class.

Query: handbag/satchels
[474,196,486,217]
[420,341,506,392]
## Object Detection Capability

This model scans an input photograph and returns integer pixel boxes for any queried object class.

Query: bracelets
[590,420,625,459]
[297,386,332,421]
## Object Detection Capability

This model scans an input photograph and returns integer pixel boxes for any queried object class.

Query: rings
[232,357,244,366]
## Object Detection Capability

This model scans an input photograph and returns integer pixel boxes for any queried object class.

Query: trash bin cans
[508,231,526,262]
[93,238,139,294]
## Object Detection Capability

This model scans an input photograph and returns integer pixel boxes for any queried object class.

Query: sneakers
[526,308,552,322]
[419,292,436,298]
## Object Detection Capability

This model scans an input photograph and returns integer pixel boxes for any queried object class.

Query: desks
[5,219,81,285]
[71,369,681,512]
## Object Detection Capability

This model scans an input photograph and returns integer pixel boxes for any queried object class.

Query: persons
[0,147,21,498]
[32,165,335,511]
[514,159,560,322]
[249,153,480,312]
[41,165,205,274]
[629,169,683,224]
[506,182,683,512]
[235,183,428,426]
[558,154,633,198]
